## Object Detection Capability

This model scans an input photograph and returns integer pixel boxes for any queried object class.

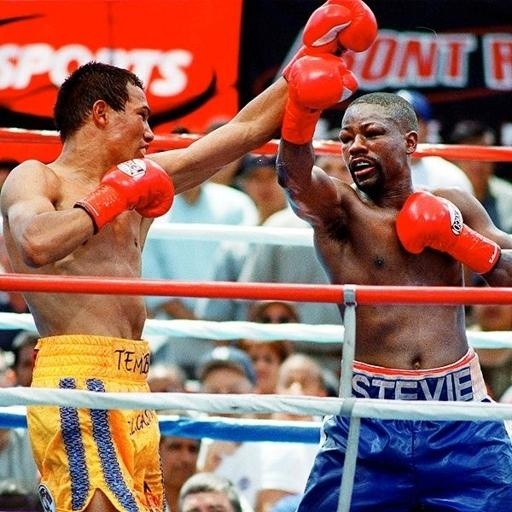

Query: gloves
[396,190,501,276]
[280,0,378,145]
[74,158,175,235]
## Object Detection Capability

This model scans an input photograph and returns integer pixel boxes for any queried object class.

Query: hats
[198,347,257,386]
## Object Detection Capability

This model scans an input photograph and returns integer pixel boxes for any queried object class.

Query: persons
[275,54,512,512]
[1,0,378,512]
